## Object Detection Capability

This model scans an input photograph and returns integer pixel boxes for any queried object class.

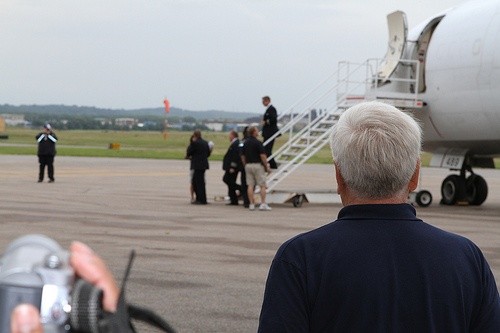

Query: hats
[44,122,51,128]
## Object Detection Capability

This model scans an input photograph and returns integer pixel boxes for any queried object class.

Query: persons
[257,100,500,333]
[222,130,246,204]
[261,96,282,169]
[185,129,214,204]
[10,240,122,332]
[35,121,58,183]
[241,126,272,210]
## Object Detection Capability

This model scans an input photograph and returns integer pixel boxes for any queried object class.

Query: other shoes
[243,204,249,207]
[48,179,54,183]
[259,203,272,210]
[249,204,255,210]
[192,200,205,204]
[37,179,43,182]
[225,203,238,205]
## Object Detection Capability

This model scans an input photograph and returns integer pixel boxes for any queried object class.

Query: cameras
[0,234,76,333]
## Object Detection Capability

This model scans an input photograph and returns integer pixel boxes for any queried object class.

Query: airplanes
[226,1,500,207]
